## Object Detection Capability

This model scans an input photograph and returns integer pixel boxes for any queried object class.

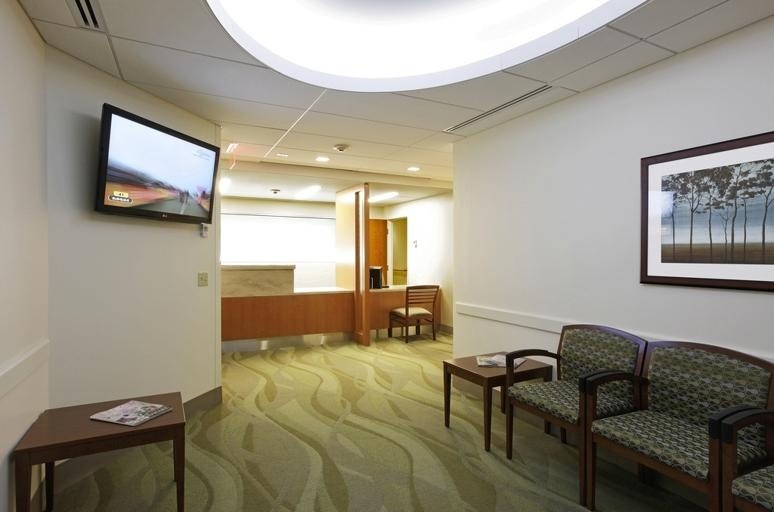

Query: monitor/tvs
[93,102,220,224]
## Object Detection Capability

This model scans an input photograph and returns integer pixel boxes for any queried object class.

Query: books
[476,353,528,368]
[89,399,173,427]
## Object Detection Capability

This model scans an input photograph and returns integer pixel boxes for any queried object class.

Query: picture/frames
[639,130,773,293]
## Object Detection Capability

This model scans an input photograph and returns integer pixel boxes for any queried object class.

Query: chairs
[388,284,440,344]
[587,341,774,512]
[721,409,774,512]
[503,323,647,509]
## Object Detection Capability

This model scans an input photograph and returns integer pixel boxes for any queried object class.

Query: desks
[11,390,188,512]
[441,351,554,452]
